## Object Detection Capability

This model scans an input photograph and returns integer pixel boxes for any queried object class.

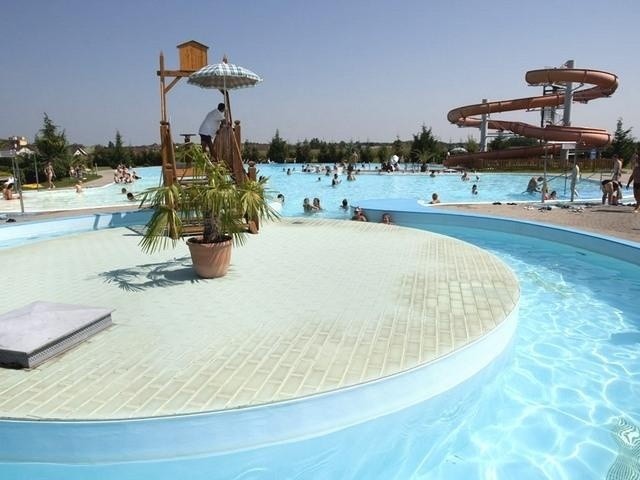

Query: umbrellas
[187,54,263,125]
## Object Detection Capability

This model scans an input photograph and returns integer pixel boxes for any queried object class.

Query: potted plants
[130,142,283,279]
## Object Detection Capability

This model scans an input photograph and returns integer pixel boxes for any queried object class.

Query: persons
[601,152,640,212]
[541,180,550,193]
[67,164,89,182]
[565,160,580,198]
[127,193,136,201]
[527,176,541,194]
[277,194,285,206]
[341,199,350,212]
[303,198,319,213]
[75,180,84,194]
[3,184,9,194]
[282,149,481,196]
[198,103,232,162]
[44,161,57,190]
[430,193,440,204]
[351,207,367,222]
[114,163,142,185]
[544,191,557,200]
[312,198,324,212]
[379,213,395,225]
[122,188,127,193]
[4,183,20,200]
